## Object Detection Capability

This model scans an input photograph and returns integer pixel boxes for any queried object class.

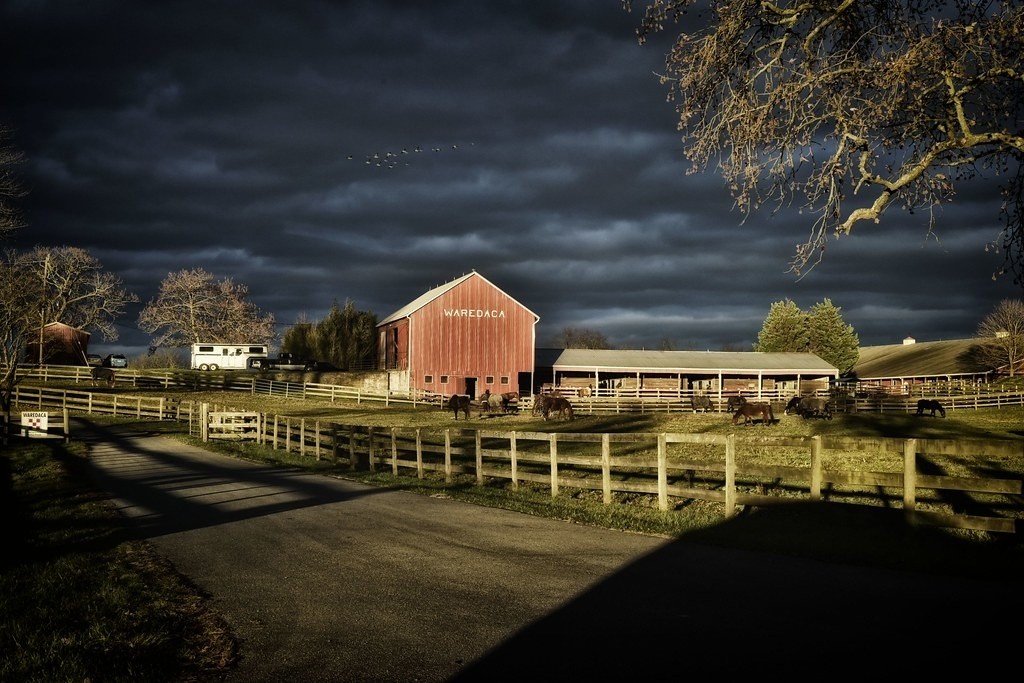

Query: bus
[191,342,269,372]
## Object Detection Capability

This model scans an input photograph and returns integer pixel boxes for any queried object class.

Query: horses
[916,399,946,418]
[579,384,593,398]
[448,389,574,422]
[89,367,116,388]
[690,396,833,427]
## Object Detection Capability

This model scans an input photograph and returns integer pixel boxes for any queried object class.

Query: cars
[87,353,103,365]
[105,353,127,368]
